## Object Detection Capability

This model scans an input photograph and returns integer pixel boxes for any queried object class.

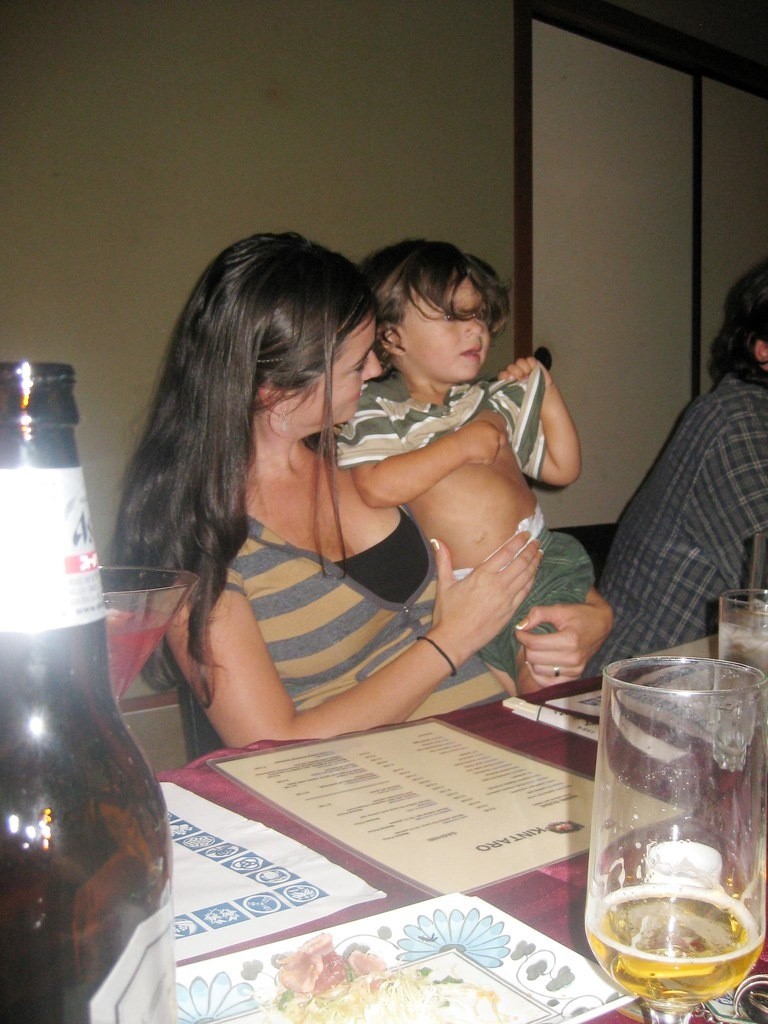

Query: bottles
[0,360,182,1024]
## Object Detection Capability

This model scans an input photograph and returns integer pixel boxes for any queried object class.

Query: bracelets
[417,635,458,676]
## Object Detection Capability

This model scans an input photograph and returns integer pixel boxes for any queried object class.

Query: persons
[108,224,615,751]
[331,236,593,696]
[584,259,768,677]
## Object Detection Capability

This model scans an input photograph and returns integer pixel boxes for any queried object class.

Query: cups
[101,565,200,704]
[748,533,768,615]
[647,841,724,888]
[713,590,768,771]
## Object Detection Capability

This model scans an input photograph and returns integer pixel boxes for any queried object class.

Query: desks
[158,666,768,1024]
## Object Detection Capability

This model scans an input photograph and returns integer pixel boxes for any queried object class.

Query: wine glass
[584,656,768,1024]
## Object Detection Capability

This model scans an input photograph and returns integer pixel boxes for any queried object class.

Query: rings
[554,666,560,677]
